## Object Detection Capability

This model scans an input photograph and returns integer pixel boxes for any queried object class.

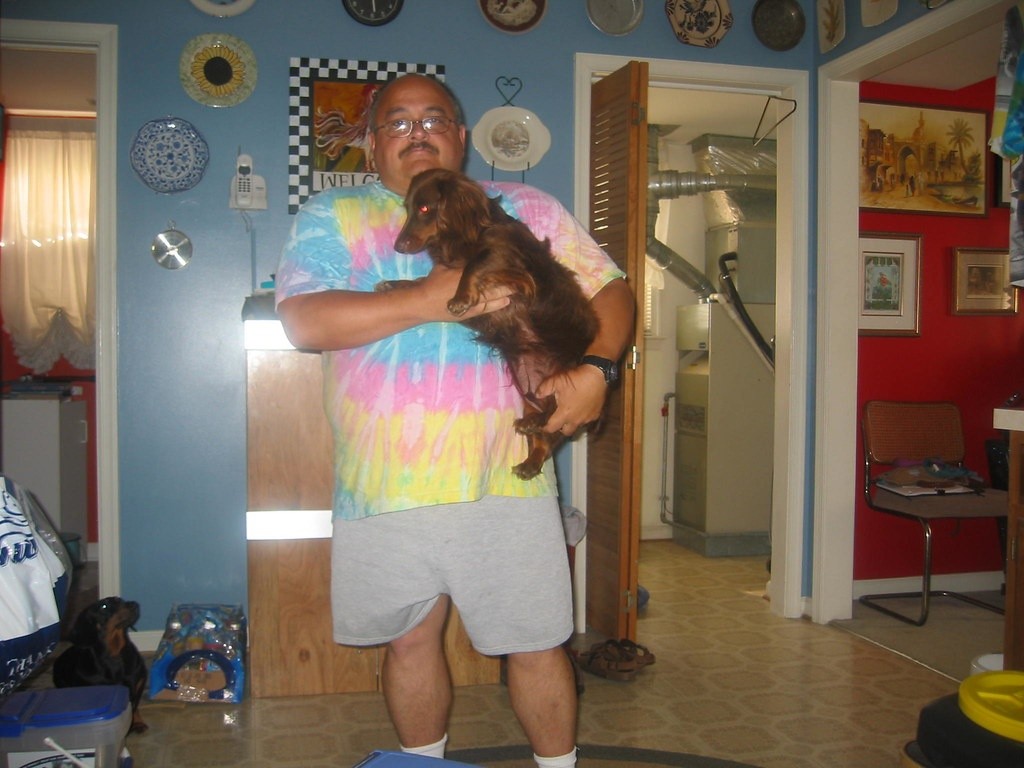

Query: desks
[993,408,1024,673]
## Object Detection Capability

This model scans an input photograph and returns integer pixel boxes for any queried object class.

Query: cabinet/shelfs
[242,296,503,700]
[0,395,88,570]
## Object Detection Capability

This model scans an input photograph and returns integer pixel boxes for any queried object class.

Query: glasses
[373,115,460,138]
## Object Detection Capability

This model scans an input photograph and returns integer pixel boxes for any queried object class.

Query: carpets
[828,589,1005,684]
[444,745,765,768]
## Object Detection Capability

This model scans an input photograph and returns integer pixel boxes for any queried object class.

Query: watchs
[581,356,619,385]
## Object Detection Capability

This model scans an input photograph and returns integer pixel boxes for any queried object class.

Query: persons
[274,73,634,768]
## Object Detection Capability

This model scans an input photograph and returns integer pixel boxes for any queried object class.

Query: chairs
[859,399,1005,626]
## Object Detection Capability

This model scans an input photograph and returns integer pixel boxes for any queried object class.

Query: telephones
[229,153,268,209]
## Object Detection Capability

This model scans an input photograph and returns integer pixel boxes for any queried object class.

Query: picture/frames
[858,97,990,218]
[858,230,925,337]
[289,56,445,215]
[996,154,1023,209]
[950,247,1019,315]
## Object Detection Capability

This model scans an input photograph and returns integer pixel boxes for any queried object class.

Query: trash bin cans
[0,685,132,768]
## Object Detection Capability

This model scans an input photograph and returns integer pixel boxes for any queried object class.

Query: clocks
[342,0,405,26]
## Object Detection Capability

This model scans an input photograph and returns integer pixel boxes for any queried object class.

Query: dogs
[55,596,150,735]
[393,169,608,480]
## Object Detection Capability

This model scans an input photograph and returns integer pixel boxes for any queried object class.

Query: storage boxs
[0,686,132,768]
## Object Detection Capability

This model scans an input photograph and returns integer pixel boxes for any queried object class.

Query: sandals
[579,639,655,681]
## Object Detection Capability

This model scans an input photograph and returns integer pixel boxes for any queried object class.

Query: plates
[665,0,734,49]
[180,32,258,108]
[477,0,548,36]
[129,116,209,194]
[584,0,644,37]
[751,0,806,51]
[188,0,256,18]
[471,105,551,171]
[816,0,846,55]
[860,0,898,28]
